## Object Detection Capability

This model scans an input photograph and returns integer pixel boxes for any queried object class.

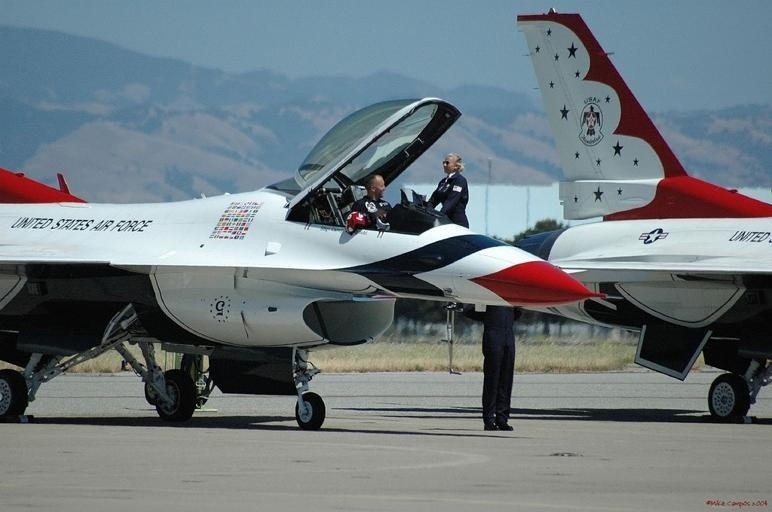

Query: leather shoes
[484,423,514,431]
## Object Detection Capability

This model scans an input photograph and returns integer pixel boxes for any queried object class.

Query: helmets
[346,211,367,235]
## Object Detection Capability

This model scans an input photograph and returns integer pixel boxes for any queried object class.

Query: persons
[352,171,393,231]
[461,301,524,431]
[425,152,469,228]
